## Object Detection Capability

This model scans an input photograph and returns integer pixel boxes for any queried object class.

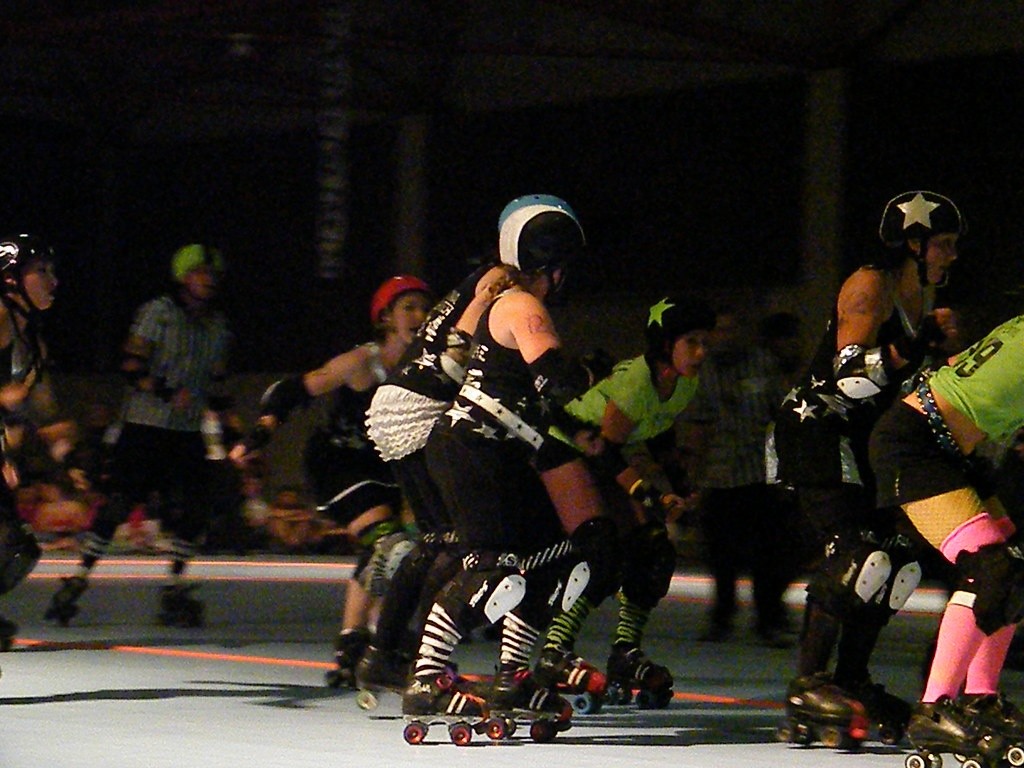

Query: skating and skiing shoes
[327,626,410,712]
[402,672,489,746]
[605,642,674,710]
[158,582,207,629]
[776,672,869,751]
[534,649,607,715]
[487,661,572,742]
[43,576,88,627]
[834,671,912,745]
[904,694,1024,768]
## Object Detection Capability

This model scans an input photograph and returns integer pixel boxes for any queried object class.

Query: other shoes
[0,615,19,652]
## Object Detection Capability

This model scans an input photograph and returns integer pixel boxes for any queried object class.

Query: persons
[709,302,809,418]
[869,314,1023,756]
[766,189,962,727]
[535,292,712,690]
[43,244,245,613]
[245,475,315,551]
[365,192,618,718]
[229,273,434,675]
[1,229,95,658]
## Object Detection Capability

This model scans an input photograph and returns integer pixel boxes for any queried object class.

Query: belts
[915,377,975,474]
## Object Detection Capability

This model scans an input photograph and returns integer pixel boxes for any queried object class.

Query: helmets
[0,233,56,278]
[498,194,587,273]
[645,296,718,353]
[879,191,968,243]
[371,275,440,326]
[169,245,224,283]
